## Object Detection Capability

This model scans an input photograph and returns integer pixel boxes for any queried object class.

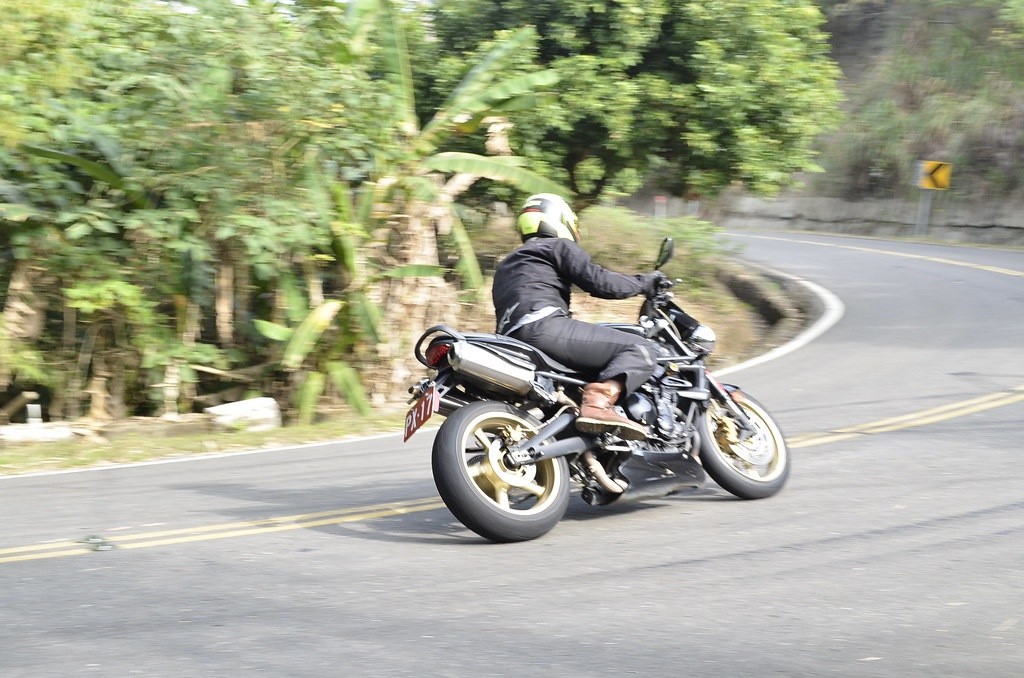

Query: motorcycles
[401,234,792,544]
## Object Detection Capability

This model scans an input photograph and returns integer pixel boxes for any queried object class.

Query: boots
[574,382,647,441]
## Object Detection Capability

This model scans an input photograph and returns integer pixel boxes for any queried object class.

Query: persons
[493,193,670,494]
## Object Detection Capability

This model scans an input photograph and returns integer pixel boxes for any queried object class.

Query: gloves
[637,270,668,299]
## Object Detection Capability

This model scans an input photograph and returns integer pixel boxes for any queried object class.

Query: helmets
[515,192,582,244]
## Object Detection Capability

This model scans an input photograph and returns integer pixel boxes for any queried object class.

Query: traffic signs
[915,160,952,191]
